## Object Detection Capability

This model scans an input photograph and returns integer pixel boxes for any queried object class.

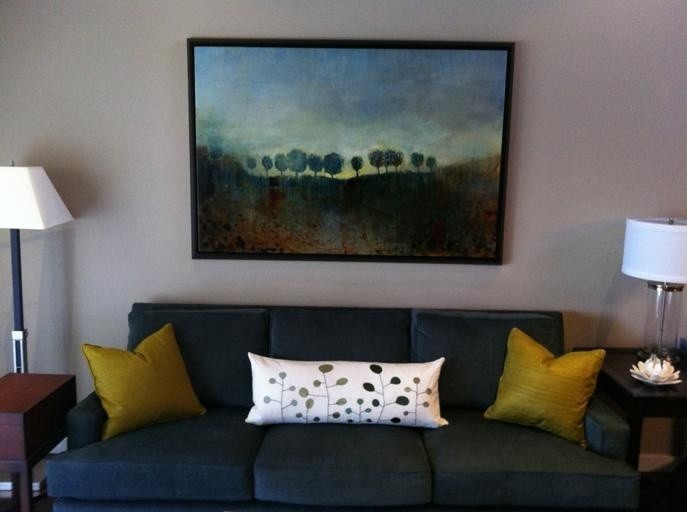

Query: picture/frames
[188,33,513,265]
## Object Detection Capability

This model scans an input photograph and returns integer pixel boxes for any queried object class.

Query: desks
[571,343,687,505]
[1,373,77,512]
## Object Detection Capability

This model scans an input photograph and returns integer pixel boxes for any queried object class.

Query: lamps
[621,215,684,364]
[1,160,75,375]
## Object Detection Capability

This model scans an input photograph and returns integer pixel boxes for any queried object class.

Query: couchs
[44,302,639,509]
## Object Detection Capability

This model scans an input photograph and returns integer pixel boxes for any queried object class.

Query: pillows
[80,320,608,456]
